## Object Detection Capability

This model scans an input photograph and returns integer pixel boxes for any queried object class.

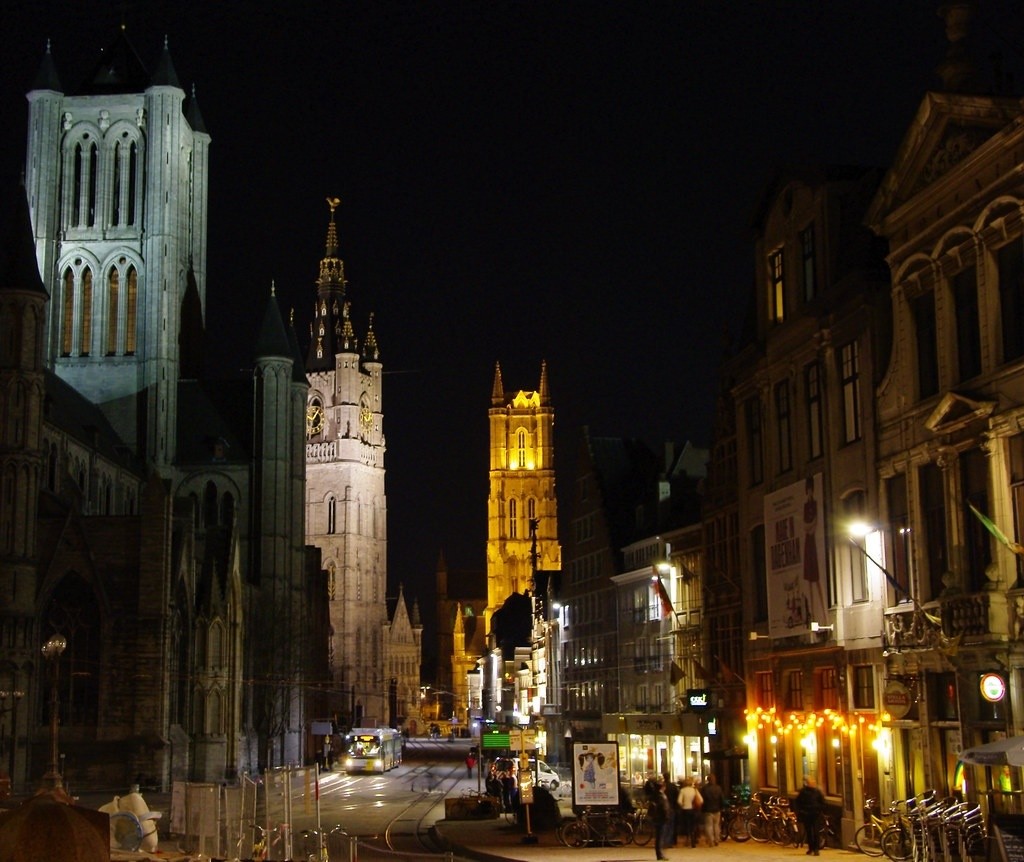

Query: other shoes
[709,841,718,847]
[658,857,668,860]
[692,840,698,848]
[806,850,819,855]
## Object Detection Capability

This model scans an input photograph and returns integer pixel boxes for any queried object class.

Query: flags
[654,577,673,618]
[669,661,685,686]
[718,658,732,682]
[694,660,715,680]
[682,565,693,585]
[970,505,1014,550]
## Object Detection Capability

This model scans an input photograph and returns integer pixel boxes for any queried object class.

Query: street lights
[41,633,67,786]
[563,717,575,815]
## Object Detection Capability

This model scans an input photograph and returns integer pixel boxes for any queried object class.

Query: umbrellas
[956,735,1024,768]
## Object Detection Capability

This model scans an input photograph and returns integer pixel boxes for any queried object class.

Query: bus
[345,728,402,775]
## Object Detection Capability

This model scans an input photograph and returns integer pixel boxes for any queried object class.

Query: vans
[491,757,560,791]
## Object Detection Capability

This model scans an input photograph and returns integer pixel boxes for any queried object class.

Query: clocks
[359,408,374,434]
[306,406,326,435]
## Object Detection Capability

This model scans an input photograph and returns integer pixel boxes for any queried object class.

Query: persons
[678,780,703,846]
[466,755,474,778]
[795,775,828,855]
[802,477,826,626]
[653,781,670,861]
[486,763,517,813]
[663,773,679,844]
[700,775,726,845]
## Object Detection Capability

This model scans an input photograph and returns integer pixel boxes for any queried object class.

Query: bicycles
[633,798,653,847]
[721,789,966,862]
[554,809,633,849]
[451,786,501,821]
[505,800,520,825]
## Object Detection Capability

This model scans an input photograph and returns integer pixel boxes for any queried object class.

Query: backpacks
[647,798,662,817]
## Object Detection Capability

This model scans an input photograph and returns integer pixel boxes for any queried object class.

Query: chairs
[904,789,987,862]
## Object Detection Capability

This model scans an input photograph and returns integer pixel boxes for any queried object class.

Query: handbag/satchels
[693,789,702,810]
